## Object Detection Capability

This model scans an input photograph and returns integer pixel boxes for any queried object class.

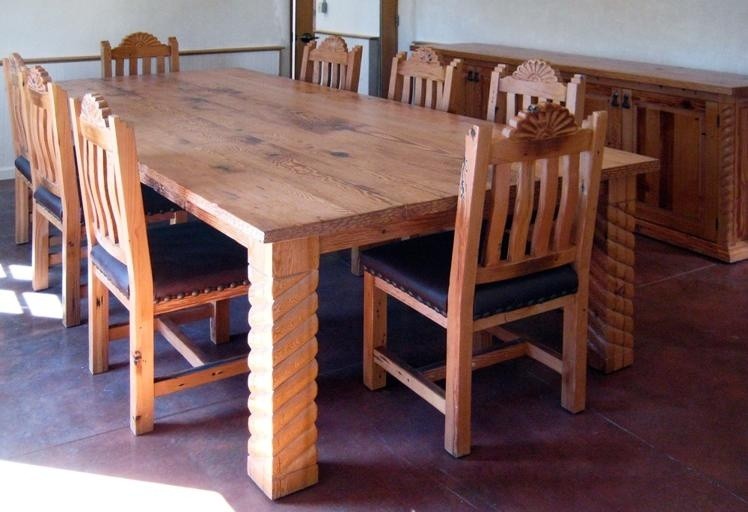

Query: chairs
[68,92,250,436]
[358,102,608,458]
[0,52,88,249]
[350,44,465,278]
[298,33,363,92]
[20,64,189,329]
[100,32,180,78]
[486,57,588,231]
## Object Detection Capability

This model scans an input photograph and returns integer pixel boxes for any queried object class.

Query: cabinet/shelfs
[564,74,747,265]
[457,52,522,125]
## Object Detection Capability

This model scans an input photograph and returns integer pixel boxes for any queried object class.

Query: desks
[53,67,660,500]
